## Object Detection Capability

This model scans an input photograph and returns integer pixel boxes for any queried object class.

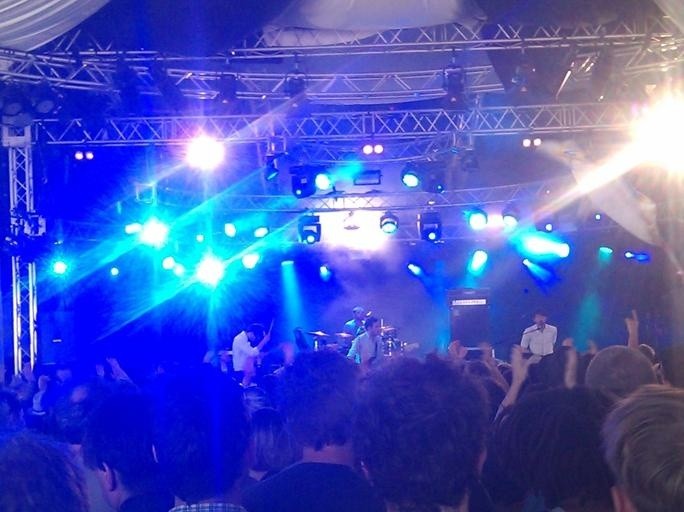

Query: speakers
[449,287,491,360]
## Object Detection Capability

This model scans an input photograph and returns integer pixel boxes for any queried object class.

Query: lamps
[261,148,521,243]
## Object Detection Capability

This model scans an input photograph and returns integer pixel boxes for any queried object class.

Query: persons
[1,308,683,510]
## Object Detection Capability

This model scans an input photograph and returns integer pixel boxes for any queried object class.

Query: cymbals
[307,331,329,337]
[333,332,353,338]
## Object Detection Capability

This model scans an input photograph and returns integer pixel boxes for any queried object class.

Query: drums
[321,343,338,353]
[383,337,402,353]
[380,327,397,338]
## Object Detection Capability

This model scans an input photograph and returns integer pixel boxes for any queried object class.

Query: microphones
[540,320,545,333]
